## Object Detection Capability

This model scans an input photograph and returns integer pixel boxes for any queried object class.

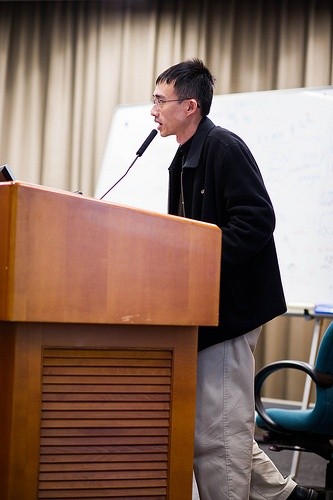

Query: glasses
[152,97,201,109]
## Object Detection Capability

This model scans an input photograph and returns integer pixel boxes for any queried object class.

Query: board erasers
[314,304,333,315]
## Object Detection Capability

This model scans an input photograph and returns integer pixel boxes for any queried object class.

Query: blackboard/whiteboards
[94,87,333,318]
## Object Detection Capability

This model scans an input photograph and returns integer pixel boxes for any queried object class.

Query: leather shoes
[288,485,318,500]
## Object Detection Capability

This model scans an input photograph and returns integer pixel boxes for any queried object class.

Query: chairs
[254,321,333,500]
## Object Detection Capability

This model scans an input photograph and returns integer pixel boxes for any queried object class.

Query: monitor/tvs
[0,165,13,182]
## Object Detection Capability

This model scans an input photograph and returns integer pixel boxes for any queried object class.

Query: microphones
[100,129,158,200]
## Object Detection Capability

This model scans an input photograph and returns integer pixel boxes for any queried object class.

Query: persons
[150,58,318,500]
